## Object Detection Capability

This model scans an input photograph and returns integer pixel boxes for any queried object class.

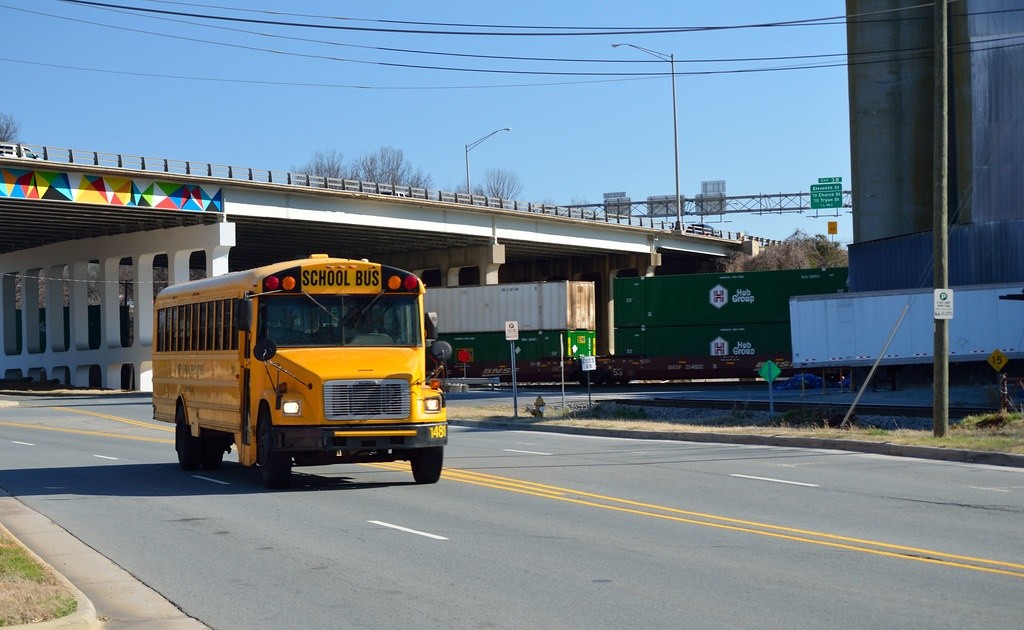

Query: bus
[151,252,452,491]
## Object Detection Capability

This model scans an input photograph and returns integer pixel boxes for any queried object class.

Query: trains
[15,265,848,384]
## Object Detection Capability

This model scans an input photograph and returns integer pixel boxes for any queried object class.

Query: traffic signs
[818,177,842,183]
[810,183,842,209]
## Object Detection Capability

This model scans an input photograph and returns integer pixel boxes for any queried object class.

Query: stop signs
[457,350,470,362]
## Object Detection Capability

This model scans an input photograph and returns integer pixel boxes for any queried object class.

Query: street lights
[465,128,510,204]
[611,43,683,230]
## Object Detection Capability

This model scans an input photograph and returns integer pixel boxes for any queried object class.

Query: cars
[0,144,43,160]
[686,223,721,236]
